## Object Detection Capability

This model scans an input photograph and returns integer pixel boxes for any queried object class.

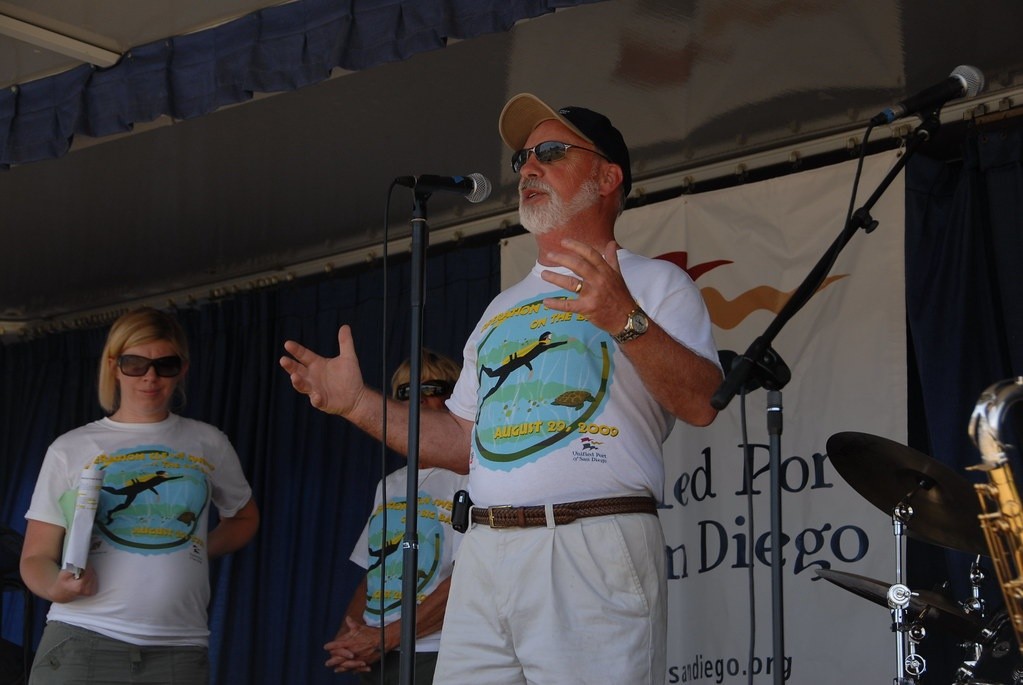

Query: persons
[279,93,725,685]
[20,307,260,685]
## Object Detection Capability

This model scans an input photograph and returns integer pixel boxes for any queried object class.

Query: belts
[471,496,659,529]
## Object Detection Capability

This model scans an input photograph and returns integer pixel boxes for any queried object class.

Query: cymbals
[814,565,997,648]
[824,428,1018,560]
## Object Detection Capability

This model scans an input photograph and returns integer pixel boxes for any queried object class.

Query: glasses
[395,379,448,402]
[510,140,611,173]
[118,354,185,378]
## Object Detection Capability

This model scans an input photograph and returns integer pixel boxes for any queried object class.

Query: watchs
[609,306,649,344]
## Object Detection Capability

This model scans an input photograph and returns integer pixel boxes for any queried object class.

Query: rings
[575,280,583,294]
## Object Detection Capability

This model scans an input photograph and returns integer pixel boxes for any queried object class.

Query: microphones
[395,173,492,203]
[870,65,985,127]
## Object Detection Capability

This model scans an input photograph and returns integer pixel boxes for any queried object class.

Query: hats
[499,93,632,197]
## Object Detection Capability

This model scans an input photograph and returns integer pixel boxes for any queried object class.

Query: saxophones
[960,374,1023,657]
